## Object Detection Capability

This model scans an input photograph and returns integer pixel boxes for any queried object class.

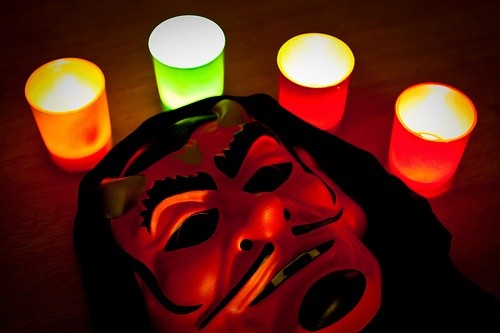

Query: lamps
[388,82,477,198]
[276,32,356,132]
[148,16,228,111]
[23,56,115,174]
